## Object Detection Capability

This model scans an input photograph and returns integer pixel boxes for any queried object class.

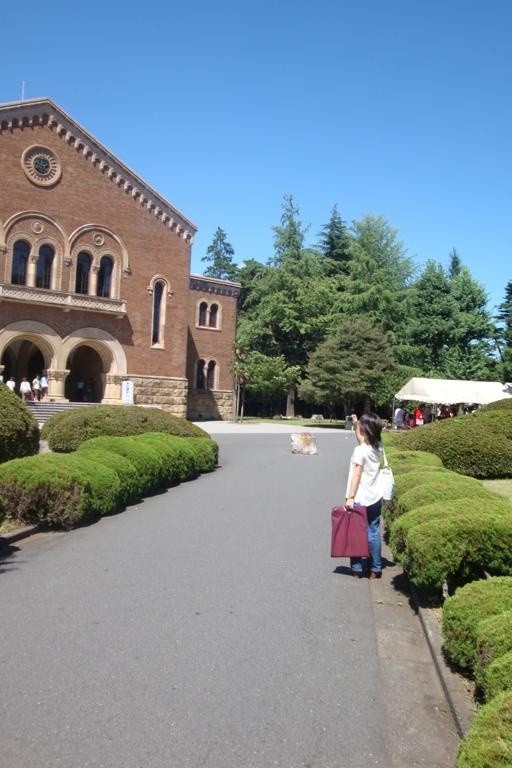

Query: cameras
[346,416,352,421]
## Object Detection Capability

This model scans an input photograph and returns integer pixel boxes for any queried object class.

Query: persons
[31,373,40,400]
[86,378,94,401]
[77,377,85,401]
[345,411,386,579]
[20,376,31,400]
[39,374,48,399]
[395,402,481,430]
[7,376,16,391]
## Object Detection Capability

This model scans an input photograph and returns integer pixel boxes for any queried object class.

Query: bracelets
[346,496,354,499]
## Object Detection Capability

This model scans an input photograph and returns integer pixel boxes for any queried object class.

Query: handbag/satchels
[331,505,369,557]
[380,466,395,500]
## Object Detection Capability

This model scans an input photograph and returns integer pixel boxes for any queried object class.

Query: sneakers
[368,571,380,579]
[351,571,361,578]
